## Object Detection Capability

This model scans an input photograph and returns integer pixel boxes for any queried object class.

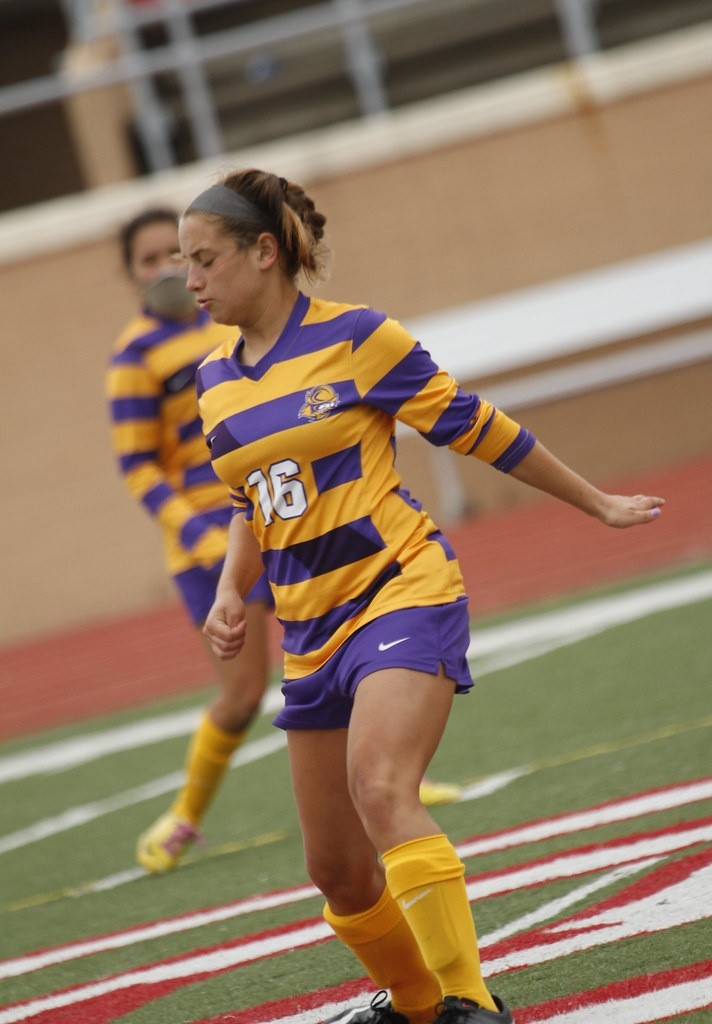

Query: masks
[142,264,202,316]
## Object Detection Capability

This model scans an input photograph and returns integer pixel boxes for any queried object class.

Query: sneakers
[349,995,512,1024]
[137,813,193,872]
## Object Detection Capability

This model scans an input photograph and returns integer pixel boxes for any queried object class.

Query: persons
[178,170,666,1024]
[105,207,460,876]
